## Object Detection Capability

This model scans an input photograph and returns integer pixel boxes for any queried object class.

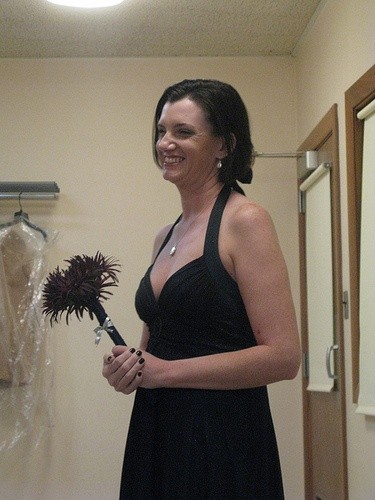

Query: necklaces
[167,184,224,256]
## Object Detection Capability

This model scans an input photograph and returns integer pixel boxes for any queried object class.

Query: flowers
[40,250,128,346]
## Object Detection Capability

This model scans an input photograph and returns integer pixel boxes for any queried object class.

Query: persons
[101,78,302,499]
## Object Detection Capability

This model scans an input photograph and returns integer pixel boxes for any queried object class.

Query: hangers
[0,191,49,245]
